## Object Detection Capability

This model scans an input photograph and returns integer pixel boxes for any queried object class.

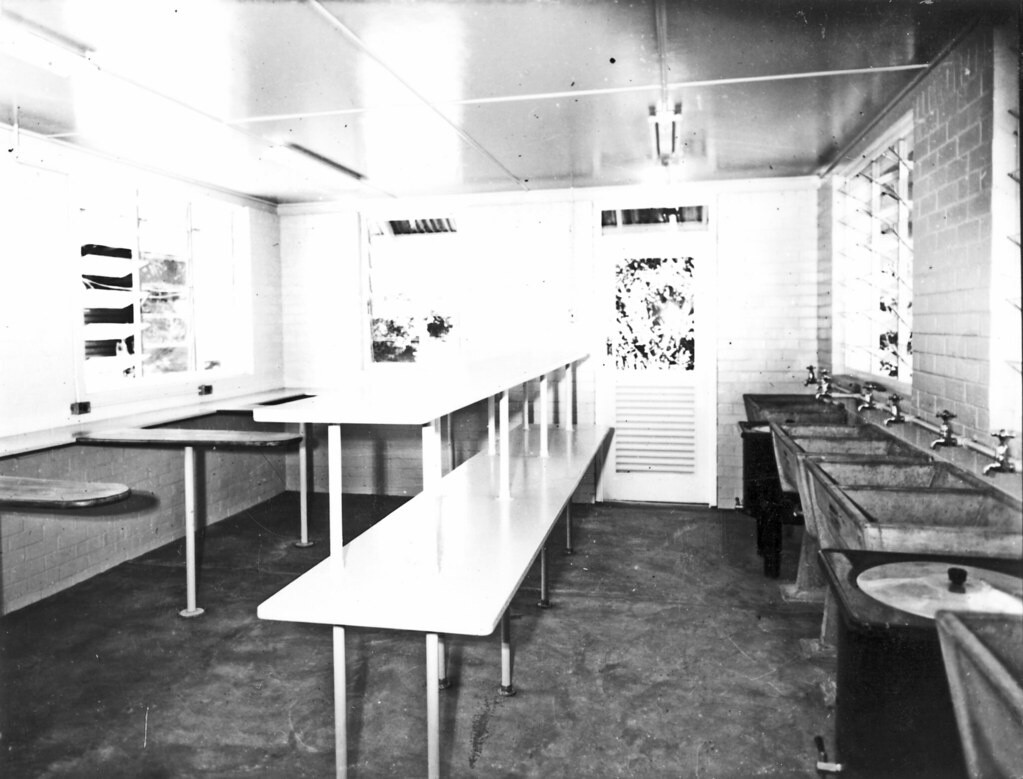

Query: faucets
[804,362,840,405]
[927,411,964,451]
[879,391,911,428]
[985,429,1021,480]
[849,380,883,414]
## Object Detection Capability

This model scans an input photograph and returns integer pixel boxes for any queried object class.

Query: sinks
[772,423,901,472]
[831,489,1023,560]
[805,461,984,534]
[784,442,934,493]
[743,393,846,427]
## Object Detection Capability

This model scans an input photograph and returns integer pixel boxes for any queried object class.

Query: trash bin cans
[738,420,805,524]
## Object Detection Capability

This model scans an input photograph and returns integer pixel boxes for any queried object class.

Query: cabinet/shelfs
[252,352,614,779]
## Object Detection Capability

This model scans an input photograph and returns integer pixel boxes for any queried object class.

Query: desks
[1,475,132,512]
[77,426,302,619]
